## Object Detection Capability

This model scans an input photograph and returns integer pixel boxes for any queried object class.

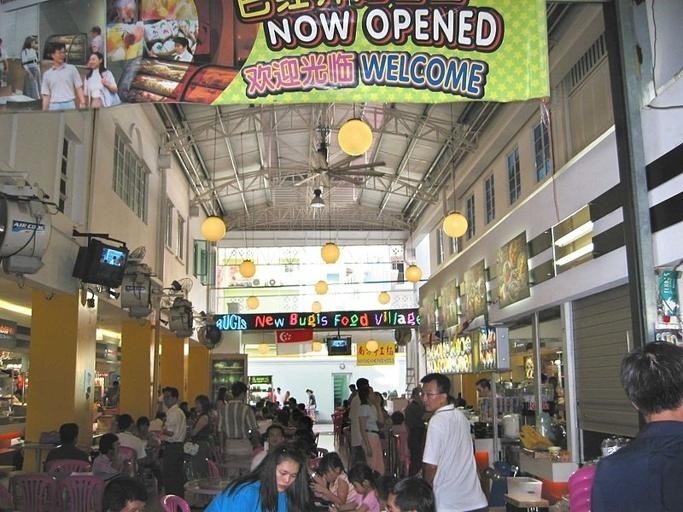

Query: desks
[184,479,233,494]
[519,450,579,506]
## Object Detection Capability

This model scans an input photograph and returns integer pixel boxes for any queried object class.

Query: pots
[500,378,521,389]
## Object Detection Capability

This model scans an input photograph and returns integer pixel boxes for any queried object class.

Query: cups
[548,446,561,463]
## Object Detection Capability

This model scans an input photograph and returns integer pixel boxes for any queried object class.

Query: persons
[589,340,683,512]
[42,374,502,511]
[110,256,116,265]
[0,26,193,112]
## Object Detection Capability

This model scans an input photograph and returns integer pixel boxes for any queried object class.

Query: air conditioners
[251,276,282,288]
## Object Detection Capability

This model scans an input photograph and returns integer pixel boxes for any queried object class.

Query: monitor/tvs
[327,339,351,355]
[478,328,511,372]
[83,239,129,288]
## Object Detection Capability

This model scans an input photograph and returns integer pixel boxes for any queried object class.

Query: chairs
[160,490,213,512]
[1,444,137,512]
[205,442,222,479]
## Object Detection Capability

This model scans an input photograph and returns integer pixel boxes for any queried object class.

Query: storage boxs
[506,477,543,502]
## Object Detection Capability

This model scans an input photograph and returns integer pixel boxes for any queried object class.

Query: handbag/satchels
[98,84,123,108]
[184,441,199,456]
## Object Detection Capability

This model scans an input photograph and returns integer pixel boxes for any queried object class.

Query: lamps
[259,329,380,356]
[247,295,259,308]
[239,132,256,278]
[378,292,390,304]
[202,106,225,241]
[312,281,328,313]
[337,104,372,155]
[309,176,340,264]
[441,103,469,239]
[405,112,422,282]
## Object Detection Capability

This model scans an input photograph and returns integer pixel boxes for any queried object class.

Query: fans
[277,103,386,186]
